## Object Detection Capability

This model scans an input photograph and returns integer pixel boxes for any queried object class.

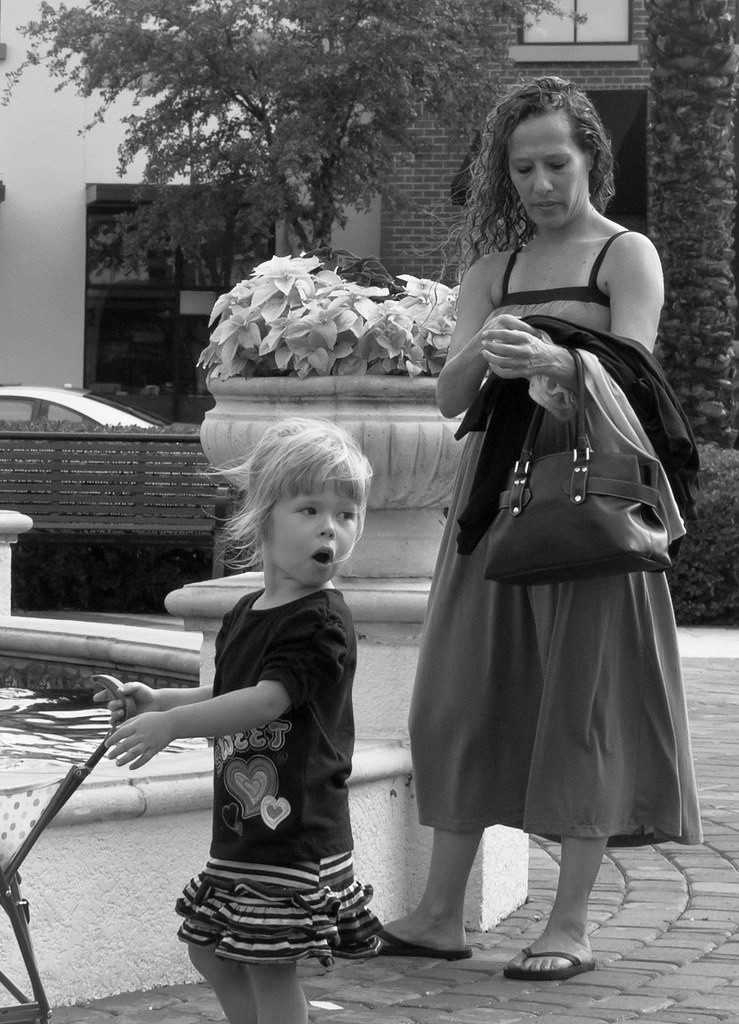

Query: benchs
[0,432,231,578]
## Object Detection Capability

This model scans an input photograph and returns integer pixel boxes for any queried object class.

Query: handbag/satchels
[481,348,675,586]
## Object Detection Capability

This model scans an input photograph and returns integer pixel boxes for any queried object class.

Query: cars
[0,381,171,434]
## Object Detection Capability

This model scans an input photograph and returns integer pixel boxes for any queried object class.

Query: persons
[374,73,704,980]
[92,419,374,1023]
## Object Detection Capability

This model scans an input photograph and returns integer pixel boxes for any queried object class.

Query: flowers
[198,244,457,377]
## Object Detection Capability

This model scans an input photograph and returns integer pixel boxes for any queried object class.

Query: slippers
[374,927,473,960]
[502,953,594,982]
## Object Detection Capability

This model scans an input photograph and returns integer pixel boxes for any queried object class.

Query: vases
[199,371,469,578]
